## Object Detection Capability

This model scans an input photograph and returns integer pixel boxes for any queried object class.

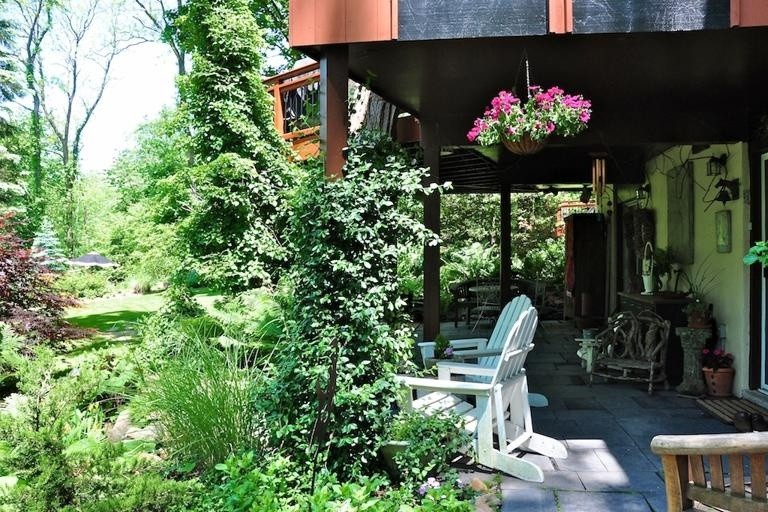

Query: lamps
[706,153,726,176]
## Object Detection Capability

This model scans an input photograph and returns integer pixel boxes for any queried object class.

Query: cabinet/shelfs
[563,212,606,320]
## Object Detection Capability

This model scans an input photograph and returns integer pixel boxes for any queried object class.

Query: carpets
[695,398,768,426]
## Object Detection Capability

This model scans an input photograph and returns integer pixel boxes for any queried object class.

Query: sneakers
[735,411,751,432]
[750,413,768,430]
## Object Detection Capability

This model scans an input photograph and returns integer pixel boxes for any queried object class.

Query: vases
[500,130,552,155]
[702,366,735,397]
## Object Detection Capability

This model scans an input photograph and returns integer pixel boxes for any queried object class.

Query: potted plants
[675,248,727,328]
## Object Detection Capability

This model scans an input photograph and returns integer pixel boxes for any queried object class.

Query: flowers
[702,347,734,373]
[466,86,593,148]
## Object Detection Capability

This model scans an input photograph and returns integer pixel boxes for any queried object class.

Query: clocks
[715,210,731,253]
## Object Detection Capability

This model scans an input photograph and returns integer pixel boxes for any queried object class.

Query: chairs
[392,294,568,483]
[449,279,536,330]
[650,430,768,511]
[587,309,671,396]
